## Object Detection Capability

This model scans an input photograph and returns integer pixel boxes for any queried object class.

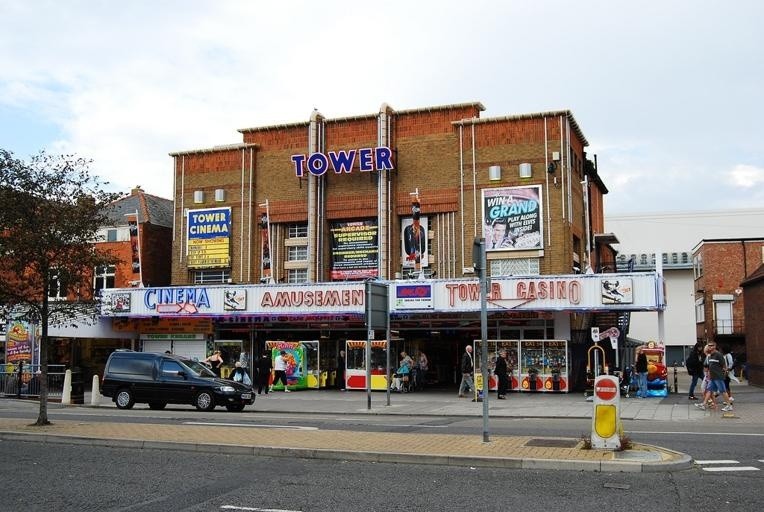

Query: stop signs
[596,379,616,401]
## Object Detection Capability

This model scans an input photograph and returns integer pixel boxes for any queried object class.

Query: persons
[228,361,243,384]
[255,351,272,394]
[202,349,223,378]
[399,351,414,391]
[337,350,346,390]
[684,340,736,411]
[489,218,513,248]
[631,346,648,398]
[416,349,428,389]
[494,347,512,399]
[268,350,291,392]
[458,344,475,397]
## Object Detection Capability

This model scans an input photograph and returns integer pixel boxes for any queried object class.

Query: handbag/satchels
[688,368,698,375]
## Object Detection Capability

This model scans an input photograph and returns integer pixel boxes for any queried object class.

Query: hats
[280,351,286,354]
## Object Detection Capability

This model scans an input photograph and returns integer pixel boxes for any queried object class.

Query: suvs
[99,347,255,413]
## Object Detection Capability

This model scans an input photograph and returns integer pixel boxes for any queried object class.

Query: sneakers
[694,403,706,410]
[721,404,733,411]
[723,397,734,404]
[689,397,697,400]
[269,389,273,392]
[285,390,290,392]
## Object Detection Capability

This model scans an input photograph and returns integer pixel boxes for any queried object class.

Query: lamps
[193,189,224,203]
